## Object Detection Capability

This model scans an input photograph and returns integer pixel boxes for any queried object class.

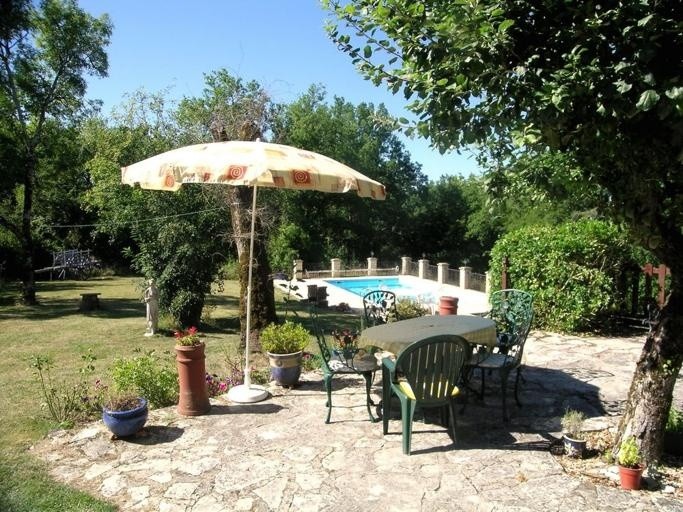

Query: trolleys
[33,248,101,281]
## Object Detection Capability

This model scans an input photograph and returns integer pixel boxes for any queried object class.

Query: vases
[439,296,458,315]
[175,343,211,416]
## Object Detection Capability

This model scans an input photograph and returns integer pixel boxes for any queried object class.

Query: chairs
[460,289,535,420]
[363,291,399,383]
[309,306,380,424]
[382,334,471,455]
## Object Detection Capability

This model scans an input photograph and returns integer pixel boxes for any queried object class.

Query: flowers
[174,325,200,346]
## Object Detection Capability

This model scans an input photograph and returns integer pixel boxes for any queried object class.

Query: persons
[143,277,159,337]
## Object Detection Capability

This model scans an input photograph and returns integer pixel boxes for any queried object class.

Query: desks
[359,315,496,424]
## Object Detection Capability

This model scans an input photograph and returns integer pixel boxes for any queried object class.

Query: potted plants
[260,320,311,389]
[103,394,149,439]
[617,435,647,491]
[664,409,683,456]
[561,406,590,459]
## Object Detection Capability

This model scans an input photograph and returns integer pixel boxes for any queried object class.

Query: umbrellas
[120,138,388,389]
